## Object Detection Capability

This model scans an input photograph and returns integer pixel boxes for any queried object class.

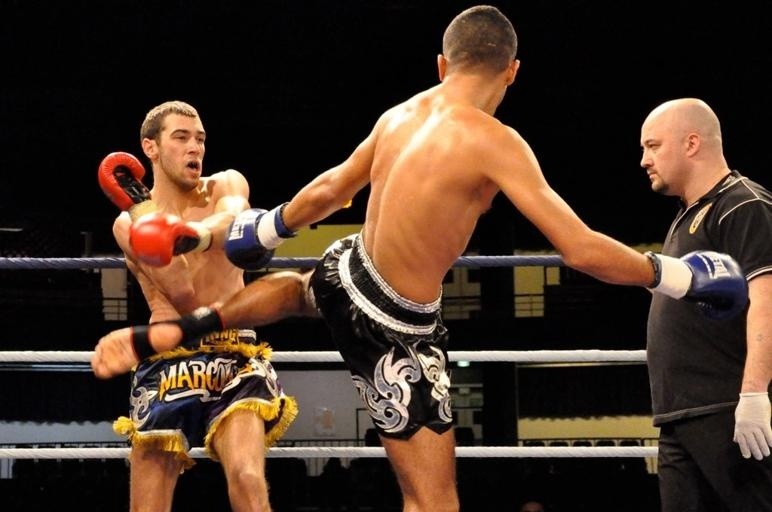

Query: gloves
[224,201,296,273]
[98,150,158,222]
[643,246,748,321]
[128,210,213,268]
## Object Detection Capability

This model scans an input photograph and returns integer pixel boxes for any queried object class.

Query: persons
[638,95,772,507]
[97,94,298,511]
[91,4,750,511]
